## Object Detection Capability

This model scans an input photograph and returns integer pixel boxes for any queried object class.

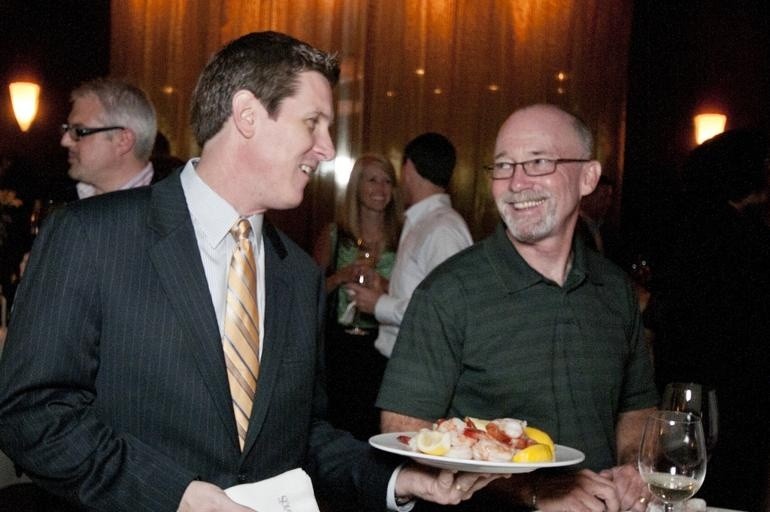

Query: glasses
[60,123,129,139]
[486,157,595,180]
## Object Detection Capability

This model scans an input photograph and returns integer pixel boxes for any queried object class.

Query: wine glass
[343,237,385,338]
[639,376,721,511]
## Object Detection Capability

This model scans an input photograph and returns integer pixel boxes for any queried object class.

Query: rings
[455,483,469,494]
[638,495,649,507]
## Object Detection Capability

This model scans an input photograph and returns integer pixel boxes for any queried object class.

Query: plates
[367,428,589,475]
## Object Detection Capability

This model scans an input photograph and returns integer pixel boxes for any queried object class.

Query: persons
[579,123,769,512]
[313,152,400,440]
[347,132,475,379]
[1,74,185,349]
[3,31,512,510]
[374,101,664,511]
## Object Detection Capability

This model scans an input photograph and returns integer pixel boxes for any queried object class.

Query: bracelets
[531,471,553,509]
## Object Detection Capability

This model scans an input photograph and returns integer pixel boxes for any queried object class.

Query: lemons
[512,443,553,463]
[417,427,451,456]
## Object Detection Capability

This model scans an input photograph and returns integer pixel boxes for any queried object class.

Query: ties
[221,218,259,454]
